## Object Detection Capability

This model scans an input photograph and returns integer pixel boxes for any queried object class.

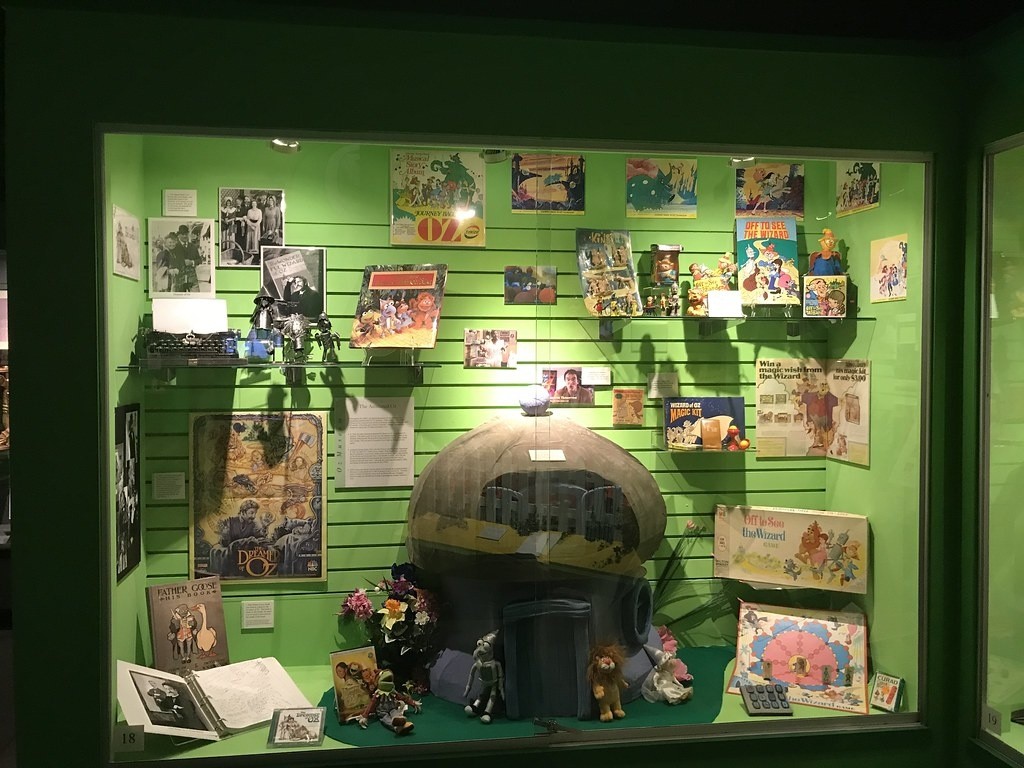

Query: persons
[253,287,341,363]
[283,275,320,314]
[221,190,281,253]
[157,225,202,293]
[162,682,208,731]
[553,369,592,403]
[146,680,166,707]
[483,330,505,368]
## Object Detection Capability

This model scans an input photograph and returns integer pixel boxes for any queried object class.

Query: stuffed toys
[357,668,422,734]
[644,644,693,704]
[463,630,505,723]
[589,645,629,721]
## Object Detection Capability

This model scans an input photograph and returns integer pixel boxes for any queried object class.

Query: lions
[587,640,628,721]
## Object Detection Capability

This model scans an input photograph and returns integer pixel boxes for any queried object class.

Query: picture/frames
[266,706,326,747]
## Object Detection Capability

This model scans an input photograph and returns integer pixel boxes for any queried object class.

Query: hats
[178,225,189,234]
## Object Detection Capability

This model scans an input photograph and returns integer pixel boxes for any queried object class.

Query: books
[117,657,315,740]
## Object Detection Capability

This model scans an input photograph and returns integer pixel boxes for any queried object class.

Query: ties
[183,243,187,248]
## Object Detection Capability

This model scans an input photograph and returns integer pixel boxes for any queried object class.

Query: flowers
[332,562,445,677]
